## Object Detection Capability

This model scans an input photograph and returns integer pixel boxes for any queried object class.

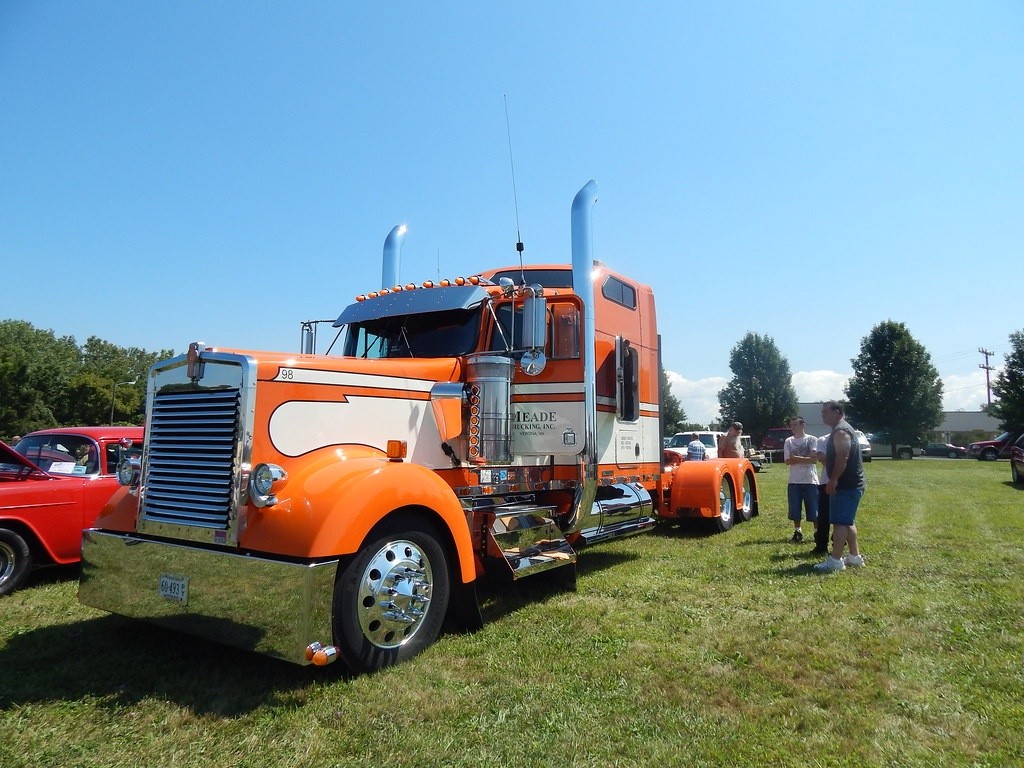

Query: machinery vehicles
[75,178,760,680]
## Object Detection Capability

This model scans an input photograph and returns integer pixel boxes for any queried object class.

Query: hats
[733,422,743,432]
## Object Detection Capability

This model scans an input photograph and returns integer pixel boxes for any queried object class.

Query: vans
[867,433,926,460]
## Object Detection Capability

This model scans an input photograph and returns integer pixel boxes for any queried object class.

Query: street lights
[109,374,137,426]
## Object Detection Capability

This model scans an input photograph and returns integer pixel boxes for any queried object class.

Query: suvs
[761,427,795,463]
[853,428,872,462]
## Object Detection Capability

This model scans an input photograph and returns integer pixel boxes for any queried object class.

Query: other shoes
[792,530,802,543]
[809,546,828,555]
[831,535,847,546]
[814,532,817,542]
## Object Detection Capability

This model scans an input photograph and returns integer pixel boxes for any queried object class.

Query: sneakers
[842,553,864,566]
[814,556,846,572]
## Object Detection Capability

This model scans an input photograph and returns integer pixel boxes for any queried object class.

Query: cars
[1009,432,1024,483]
[968,430,1018,462]
[660,430,768,474]
[925,443,967,459]
[1,426,146,594]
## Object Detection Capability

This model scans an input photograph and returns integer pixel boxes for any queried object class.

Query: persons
[813,401,866,570]
[784,417,819,542]
[74,444,96,474]
[810,433,847,554]
[718,422,744,458]
[687,433,706,461]
